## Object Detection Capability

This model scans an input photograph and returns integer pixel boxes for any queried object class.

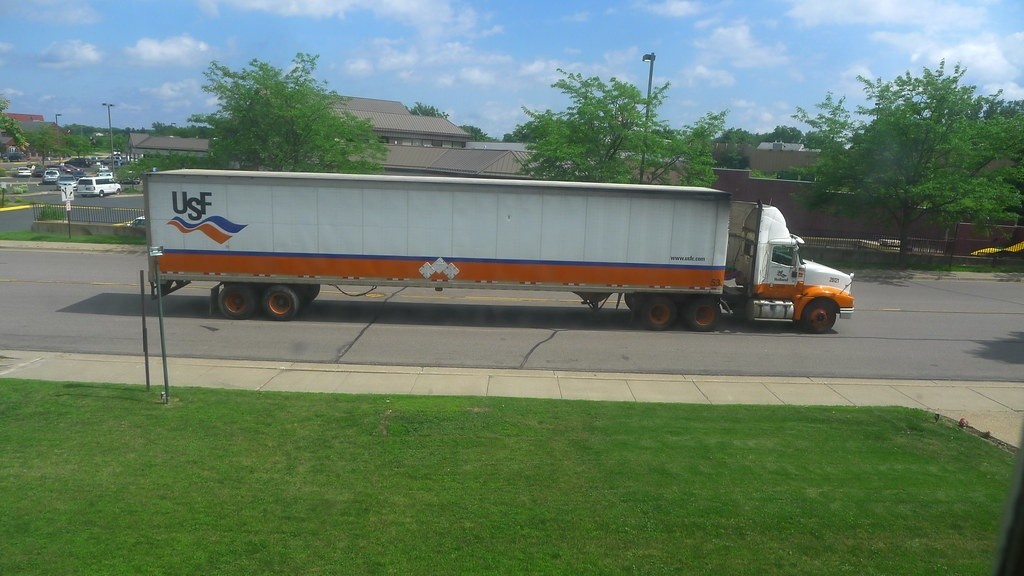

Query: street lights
[55,113,62,164]
[102,102,115,179]
[638,51,658,184]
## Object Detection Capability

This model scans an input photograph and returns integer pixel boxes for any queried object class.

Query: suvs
[42,169,60,185]
[64,157,92,168]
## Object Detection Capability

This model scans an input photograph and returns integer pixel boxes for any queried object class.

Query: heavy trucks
[143,169,855,334]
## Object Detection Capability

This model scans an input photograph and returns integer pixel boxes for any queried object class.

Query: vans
[2,152,22,163]
[77,176,122,198]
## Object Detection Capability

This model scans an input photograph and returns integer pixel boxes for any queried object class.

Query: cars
[110,216,146,228]
[34,163,85,178]
[93,154,128,177]
[55,173,78,191]
[16,166,32,178]
[119,176,140,185]
